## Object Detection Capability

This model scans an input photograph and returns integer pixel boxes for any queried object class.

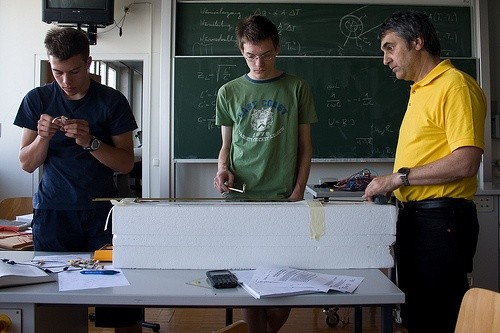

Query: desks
[305,183,395,205]
[0,250,408,333]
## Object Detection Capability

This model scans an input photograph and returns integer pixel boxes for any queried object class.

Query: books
[0,218,34,252]
[0,258,57,289]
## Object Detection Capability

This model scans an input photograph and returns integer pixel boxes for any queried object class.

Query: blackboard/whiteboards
[170,1,484,164]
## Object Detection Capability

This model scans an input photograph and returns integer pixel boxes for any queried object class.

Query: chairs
[454,288,500,333]
[0,196,33,221]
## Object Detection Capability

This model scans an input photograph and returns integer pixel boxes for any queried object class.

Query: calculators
[206,269,239,289]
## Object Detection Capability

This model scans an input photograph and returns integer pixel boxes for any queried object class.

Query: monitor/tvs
[42,0,114,26]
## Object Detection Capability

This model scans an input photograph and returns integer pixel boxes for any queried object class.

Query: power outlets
[475,196,494,213]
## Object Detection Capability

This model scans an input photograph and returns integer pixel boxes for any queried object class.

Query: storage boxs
[111,202,399,269]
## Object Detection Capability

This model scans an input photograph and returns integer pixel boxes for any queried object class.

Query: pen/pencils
[226,184,245,193]
[80,269,121,275]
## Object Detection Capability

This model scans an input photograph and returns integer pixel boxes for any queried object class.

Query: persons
[14,26,138,252]
[213,15,319,333]
[361,12,488,333]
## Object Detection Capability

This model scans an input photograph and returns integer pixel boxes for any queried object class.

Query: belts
[396,198,473,209]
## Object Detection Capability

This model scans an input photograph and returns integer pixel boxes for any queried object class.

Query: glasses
[242,50,277,62]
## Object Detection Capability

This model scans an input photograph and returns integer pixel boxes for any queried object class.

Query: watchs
[83,135,101,151]
[397,167,410,187]
